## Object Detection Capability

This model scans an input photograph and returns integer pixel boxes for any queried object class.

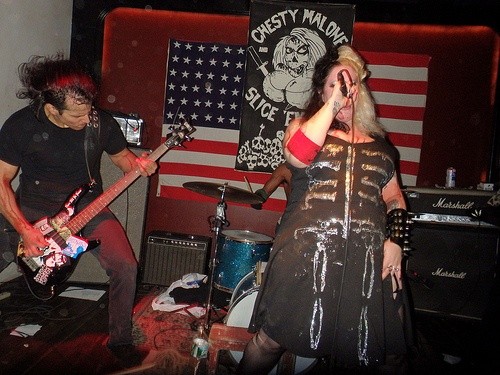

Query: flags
[155,38,432,211]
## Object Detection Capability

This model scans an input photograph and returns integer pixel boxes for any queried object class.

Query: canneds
[445,167,456,188]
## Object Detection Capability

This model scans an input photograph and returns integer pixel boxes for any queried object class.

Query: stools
[208,324,297,375]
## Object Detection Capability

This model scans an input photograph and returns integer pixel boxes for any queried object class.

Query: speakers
[399,214,500,323]
[140,230,211,287]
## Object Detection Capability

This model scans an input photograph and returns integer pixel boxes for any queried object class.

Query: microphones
[337,72,352,98]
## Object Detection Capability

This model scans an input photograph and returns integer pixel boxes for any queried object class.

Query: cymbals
[181,181,263,208]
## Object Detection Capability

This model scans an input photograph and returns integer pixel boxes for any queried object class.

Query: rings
[387,266,399,271]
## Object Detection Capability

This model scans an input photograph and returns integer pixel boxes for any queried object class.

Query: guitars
[17,120,196,289]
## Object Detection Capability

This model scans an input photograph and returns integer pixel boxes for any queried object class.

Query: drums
[221,266,318,375]
[208,228,274,293]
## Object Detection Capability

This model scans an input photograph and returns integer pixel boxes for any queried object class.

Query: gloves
[251,189,269,210]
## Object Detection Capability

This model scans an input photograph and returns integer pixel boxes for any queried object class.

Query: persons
[251,163,293,236]
[0,49,158,365]
[236,45,414,375]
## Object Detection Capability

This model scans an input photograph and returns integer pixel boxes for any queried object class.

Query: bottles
[188,325,209,375]
[445,166,456,189]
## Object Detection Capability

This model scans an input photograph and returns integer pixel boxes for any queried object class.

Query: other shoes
[107,343,140,360]
